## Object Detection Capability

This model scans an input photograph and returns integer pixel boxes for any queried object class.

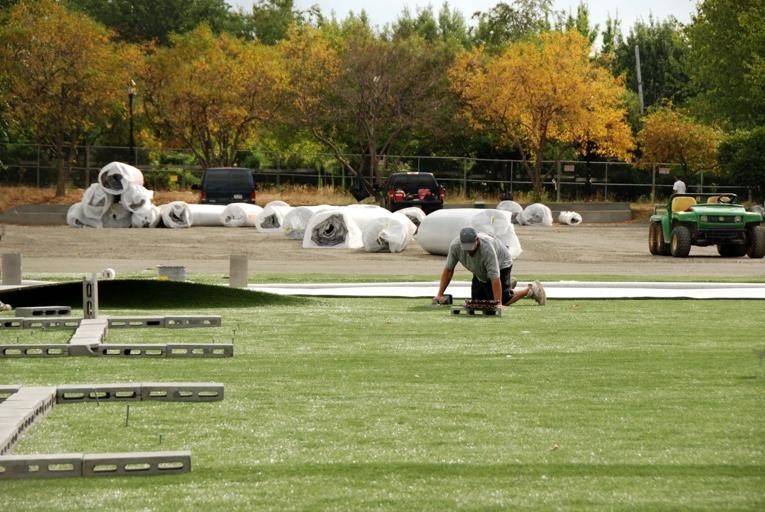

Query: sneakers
[527,281,545,306]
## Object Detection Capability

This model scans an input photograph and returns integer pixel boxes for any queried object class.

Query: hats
[460,228,477,250]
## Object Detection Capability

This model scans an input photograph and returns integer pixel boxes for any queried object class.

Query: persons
[672,174,687,194]
[432,227,546,312]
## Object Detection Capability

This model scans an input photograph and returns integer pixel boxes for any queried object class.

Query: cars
[431,161,499,195]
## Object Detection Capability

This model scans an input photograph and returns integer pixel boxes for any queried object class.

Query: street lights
[127,79,136,167]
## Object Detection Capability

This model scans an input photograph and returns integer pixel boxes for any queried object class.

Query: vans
[375,172,447,216]
[190,166,259,206]
